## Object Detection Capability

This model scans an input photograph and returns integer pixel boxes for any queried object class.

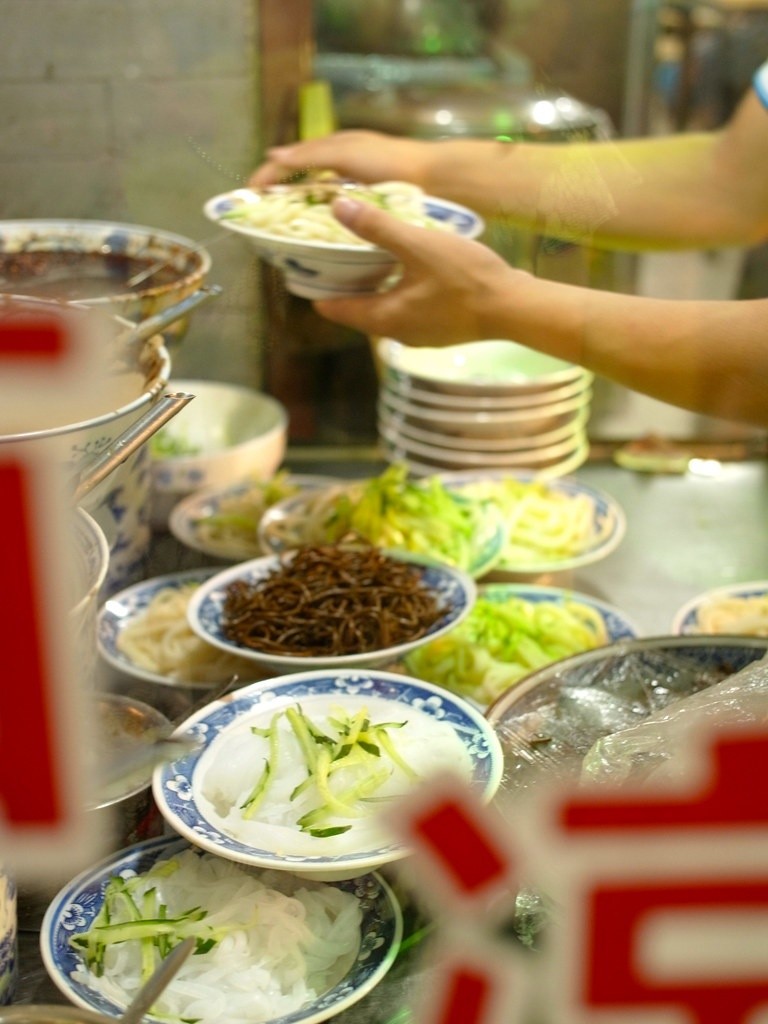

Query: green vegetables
[193,460,606,699]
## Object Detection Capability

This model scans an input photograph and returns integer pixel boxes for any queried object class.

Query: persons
[250,56,768,431]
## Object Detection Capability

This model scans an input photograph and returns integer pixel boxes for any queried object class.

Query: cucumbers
[66,862,259,1024]
[233,703,419,838]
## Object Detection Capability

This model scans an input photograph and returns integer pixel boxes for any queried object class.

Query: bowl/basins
[1,177,767,1022]
